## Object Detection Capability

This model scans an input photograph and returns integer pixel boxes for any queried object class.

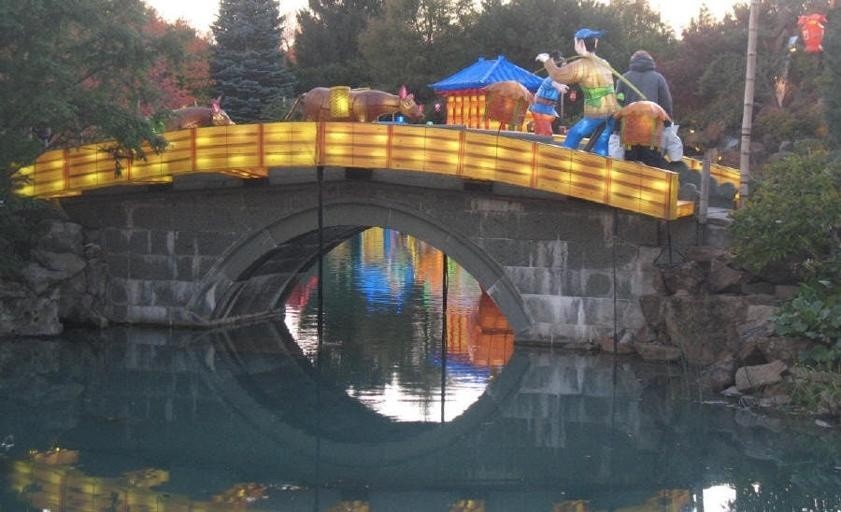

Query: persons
[529,27,616,160]
[614,49,672,164]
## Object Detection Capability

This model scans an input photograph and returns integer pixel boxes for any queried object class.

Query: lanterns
[797,11,830,53]
